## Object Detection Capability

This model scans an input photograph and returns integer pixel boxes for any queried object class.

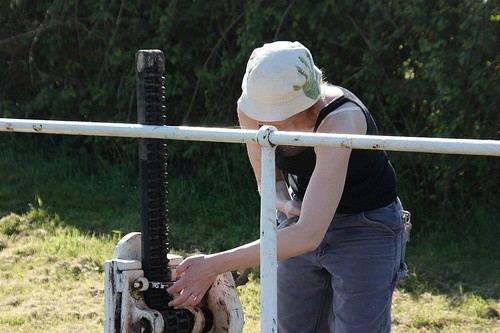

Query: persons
[163,38,414,333]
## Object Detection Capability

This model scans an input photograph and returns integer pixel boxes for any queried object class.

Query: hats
[238,40,323,124]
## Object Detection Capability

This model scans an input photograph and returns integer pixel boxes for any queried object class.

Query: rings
[191,294,197,300]
